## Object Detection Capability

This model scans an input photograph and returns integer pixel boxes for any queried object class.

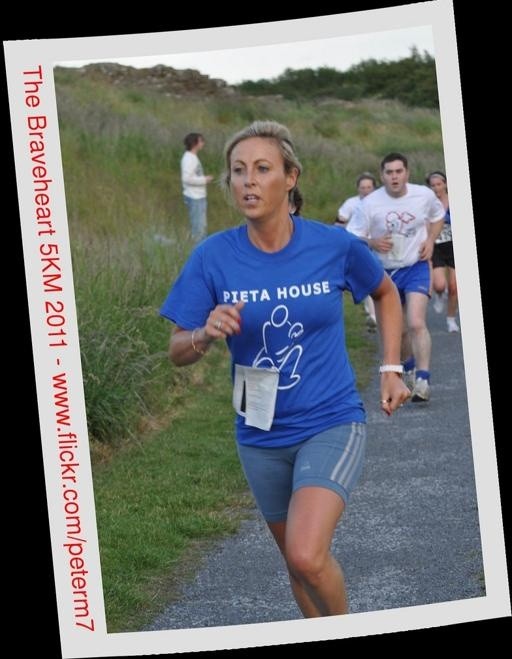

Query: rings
[400,401,405,408]
[215,319,222,329]
[381,400,390,404]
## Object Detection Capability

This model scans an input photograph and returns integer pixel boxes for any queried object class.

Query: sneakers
[446,316,458,333]
[411,377,430,402]
[402,368,416,393]
[366,316,377,336]
[434,292,445,314]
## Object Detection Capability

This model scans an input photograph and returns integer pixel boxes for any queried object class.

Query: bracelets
[379,365,405,377]
[191,328,207,355]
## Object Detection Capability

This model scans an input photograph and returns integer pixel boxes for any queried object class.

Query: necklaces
[250,216,293,250]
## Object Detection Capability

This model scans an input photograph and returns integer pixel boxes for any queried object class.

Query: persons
[160,119,415,621]
[178,131,214,237]
[289,188,303,218]
[347,153,447,402]
[335,172,376,333]
[423,170,459,334]
